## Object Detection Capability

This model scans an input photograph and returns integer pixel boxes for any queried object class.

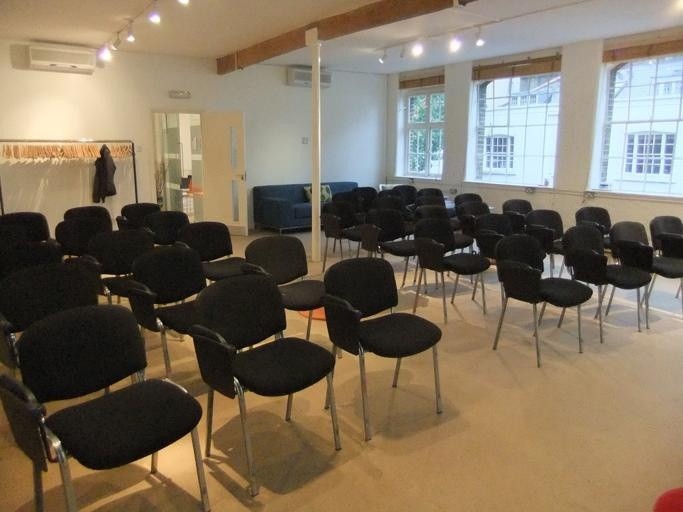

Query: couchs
[250,179,361,237]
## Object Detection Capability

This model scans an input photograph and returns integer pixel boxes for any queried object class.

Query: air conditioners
[21,42,99,76]
[283,62,335,89]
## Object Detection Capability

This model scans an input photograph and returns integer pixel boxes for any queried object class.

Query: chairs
[595,220,683,329]
[450,211,517,317]
[359,207,442,294]
[190,271,343,497]
[523,209,565,277]
[412,194,478,304]
[331,190,368,253]
[0,260,102,365]
[573,204,612,235]
[412,186,445,206]
[378,188,406,194]
[321,199,364,271]
[0,303,213,512]
[244,234,329,342]
[102,242,207,378]
[391,183,418,196]
[640,215,683,314]
[371,194,409,205]
[144,210,191,246]
[55,203,114,259]
[410,218,486,322]
[1,235,64,263]
[318,257,444,441]
[455,199,494,254]
[352,186,377,201]
[343,206,408,287]
[531,222,652,345]
[489,232,594,369]
[0,210,52,242]
[500,197,533,218]
[174,220,247,282]
[115,197,162,230]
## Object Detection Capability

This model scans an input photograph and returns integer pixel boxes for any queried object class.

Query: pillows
[304,183,334,205]
